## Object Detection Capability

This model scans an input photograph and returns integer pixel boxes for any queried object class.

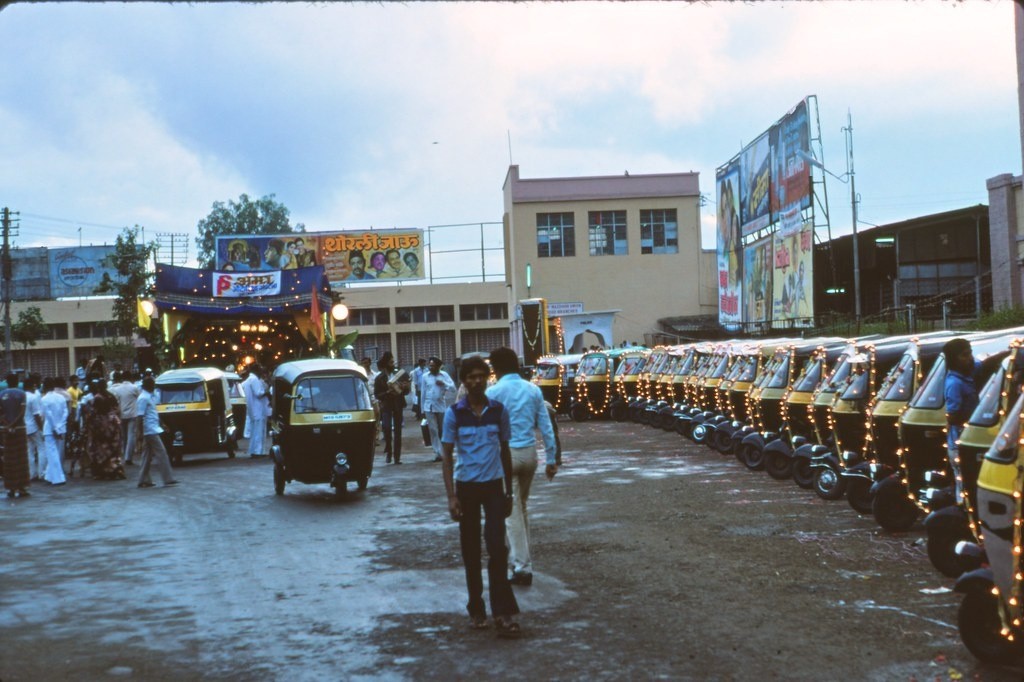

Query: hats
[429,357,442,367]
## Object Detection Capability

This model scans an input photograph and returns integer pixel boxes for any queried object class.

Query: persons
[344,249,424,281]
[719,178,742,290]
[221,238,317,272]
[941,339,1013,504]
[0,349,565,632]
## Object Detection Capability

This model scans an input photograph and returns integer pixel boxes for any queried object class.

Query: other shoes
[395,461,402,464]
[161,481,181,487]
[494,616,522,638]
[138,483,156,487]
[386,453,391,463]
[471,616,489,628]
[508,573,532,585]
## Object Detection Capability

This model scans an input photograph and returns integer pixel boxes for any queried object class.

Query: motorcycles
[146,365,240,468]
[529,325,1024,667]
[267,355,378,500]
[221,373,247,432]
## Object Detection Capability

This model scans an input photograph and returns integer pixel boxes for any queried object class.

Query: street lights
[795,148,865,338]
